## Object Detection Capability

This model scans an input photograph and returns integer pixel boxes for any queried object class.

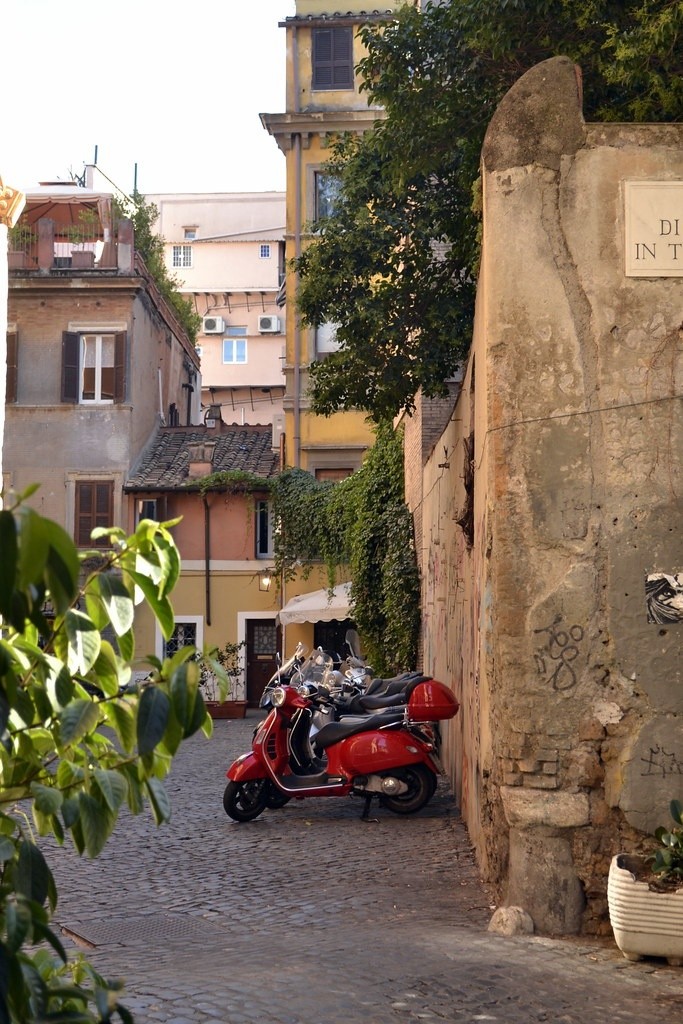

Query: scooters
[222,629,460,824]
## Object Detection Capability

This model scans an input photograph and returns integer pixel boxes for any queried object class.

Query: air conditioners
[202,316,226,335]
[258,314,283,335]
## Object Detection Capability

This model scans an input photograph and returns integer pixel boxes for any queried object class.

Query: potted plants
[5,211,39,269]
[605,799,683,968]
[66,205,100,269]
[194,638,253,718]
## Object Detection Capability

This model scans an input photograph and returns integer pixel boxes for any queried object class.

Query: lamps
[257,565,282,592]
